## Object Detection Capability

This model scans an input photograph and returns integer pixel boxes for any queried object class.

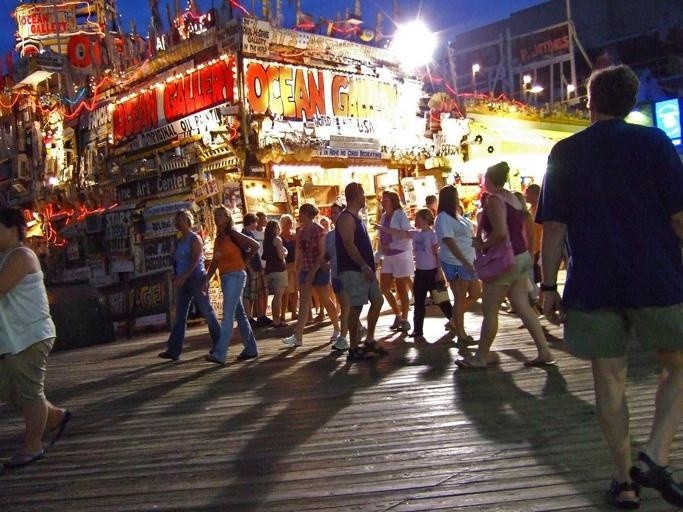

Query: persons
[1,207,71,467]
[533,64,683,510]
[256,183,390,361]
[158,204,259,365]
[371,161,555,370]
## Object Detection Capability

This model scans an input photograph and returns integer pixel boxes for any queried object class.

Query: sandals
[525,357,556,368]
[454,358,487,369]
[346,347,374,361]
[331,335,340,343]
[629,450,683,509]
[364,340,389,355]
[281,335,302,347]
[607,474,643,510]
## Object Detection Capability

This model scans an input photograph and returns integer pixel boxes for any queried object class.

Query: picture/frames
[241,178,292,216]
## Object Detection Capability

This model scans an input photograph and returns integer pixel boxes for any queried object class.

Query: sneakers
[248,313,413,333]
[157,345,183,361]
[204,354,225,365]
[209,343,215,355]
[332,336,349,351]
[235,349,255,361]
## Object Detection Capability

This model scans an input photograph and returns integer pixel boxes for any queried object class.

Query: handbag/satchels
[474,194,515,283]
[431,279,450,307]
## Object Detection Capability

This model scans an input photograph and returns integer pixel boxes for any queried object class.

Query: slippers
[3,446,45,469]
[48,408,72,449]
